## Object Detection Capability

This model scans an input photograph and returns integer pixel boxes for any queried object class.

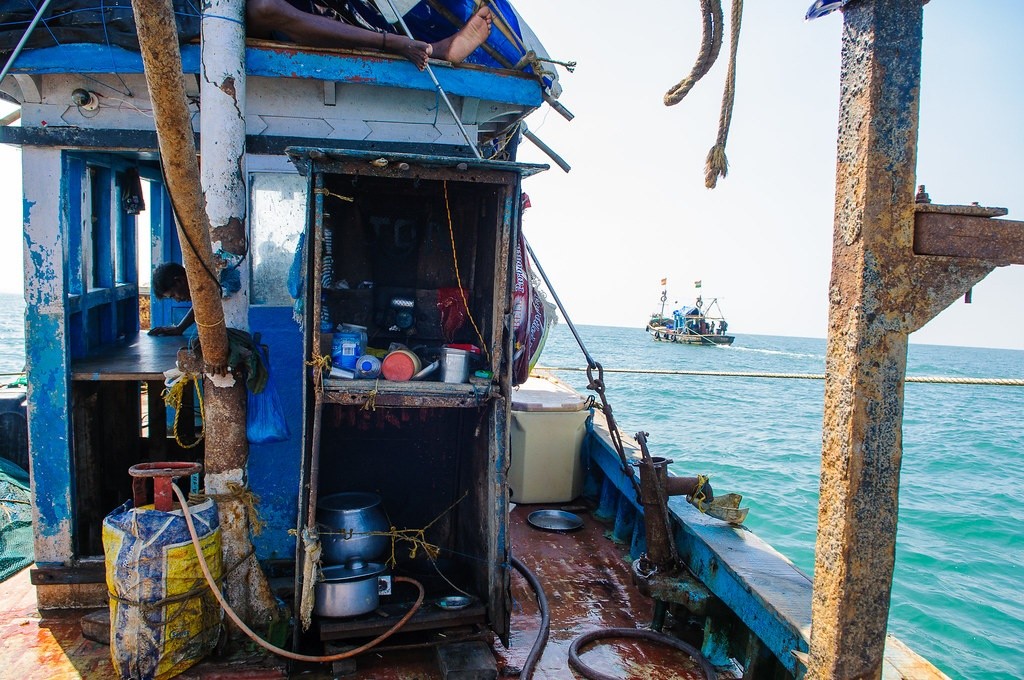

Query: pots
[315,557,386,617]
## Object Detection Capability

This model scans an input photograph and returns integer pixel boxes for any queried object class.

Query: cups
[439,348,470,383]
[327,361,354,380]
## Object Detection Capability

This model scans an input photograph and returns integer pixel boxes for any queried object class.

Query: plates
[411,360,439,381]
[435,596,470,610]
[527,509,584,530]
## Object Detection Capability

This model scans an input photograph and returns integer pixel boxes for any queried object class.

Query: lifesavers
[660,295,667,302]
[695,301,704,307]
[663,334,668,339]
[671,335,676,341]
[645,325,649,332]
[655,332,659,339]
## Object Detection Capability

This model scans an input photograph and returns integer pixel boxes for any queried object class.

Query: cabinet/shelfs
[285,144,550,680]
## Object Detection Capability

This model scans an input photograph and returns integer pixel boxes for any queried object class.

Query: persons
[244,0,492,73]
[148,261,196,337]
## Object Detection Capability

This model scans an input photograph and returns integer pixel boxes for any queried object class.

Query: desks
[69,329,205,463]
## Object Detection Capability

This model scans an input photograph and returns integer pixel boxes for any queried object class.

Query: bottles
[320,299,335,334]
[316,492,391,566]
[356,355,381,378]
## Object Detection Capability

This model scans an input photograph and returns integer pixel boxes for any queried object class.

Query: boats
[645,277,735,346]
